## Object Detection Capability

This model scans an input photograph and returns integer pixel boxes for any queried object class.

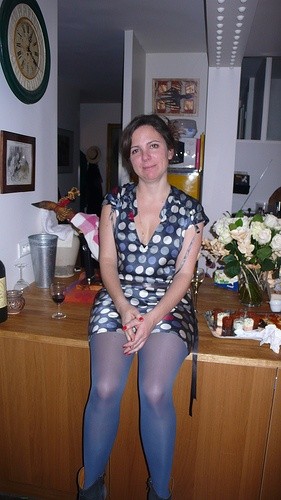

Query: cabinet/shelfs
[0,269,281,500]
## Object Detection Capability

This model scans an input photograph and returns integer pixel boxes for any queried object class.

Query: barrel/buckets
[29,234,58,288]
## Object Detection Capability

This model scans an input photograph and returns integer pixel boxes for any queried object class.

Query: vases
[238,264,264,306]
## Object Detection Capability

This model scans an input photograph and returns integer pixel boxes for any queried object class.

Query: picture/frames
[167,167,202,204]
[0,130,36,194]
[151,77,199,116]
[58,128,75,174]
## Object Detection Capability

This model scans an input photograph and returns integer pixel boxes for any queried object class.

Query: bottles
[7,289,26,314]
[0,260,8,323]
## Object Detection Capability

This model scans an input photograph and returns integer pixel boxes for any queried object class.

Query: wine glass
[51,282,67,319]
[190,266,205,317]
[13,262,33,302]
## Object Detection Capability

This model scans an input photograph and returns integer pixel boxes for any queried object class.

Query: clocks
[0,0,51,104]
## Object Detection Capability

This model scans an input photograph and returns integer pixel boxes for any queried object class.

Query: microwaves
[168,138,196,168]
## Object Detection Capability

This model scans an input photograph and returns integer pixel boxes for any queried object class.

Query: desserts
[212,308,281,331]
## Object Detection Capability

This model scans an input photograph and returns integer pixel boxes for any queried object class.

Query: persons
[77,114,210,500]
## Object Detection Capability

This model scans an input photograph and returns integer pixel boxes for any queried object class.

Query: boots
[147,476,175,500]
[77,466,107,500]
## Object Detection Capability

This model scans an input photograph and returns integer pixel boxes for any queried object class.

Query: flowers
[202,212,281,278]
